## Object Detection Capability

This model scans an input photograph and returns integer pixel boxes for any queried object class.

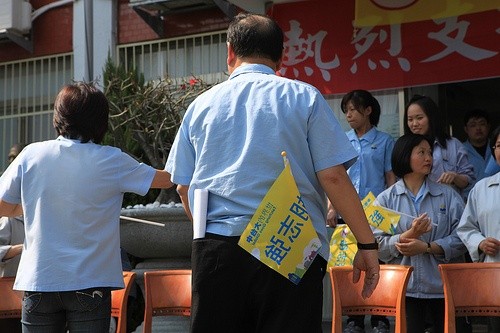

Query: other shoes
[372,321,389,333]
[343,320,363,333]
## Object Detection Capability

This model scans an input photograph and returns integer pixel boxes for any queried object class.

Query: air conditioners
[0,0,33,32]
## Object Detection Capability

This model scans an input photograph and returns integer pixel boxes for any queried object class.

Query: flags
[237,158,322,285]
[326,223,361,274]
[360,191,402,236]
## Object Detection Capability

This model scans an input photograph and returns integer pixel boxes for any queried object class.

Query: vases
[119,204,193,259]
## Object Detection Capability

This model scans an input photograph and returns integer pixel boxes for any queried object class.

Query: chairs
[0,276,25,319]
[439,262,500,333]
[144,269,192,333]
[111,272,136,333]
[329,264,413,333]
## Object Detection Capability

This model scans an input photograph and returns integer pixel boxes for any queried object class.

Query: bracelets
[357,238,379,250]
[426,243,433,254]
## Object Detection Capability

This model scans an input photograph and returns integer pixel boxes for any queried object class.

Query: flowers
[103,72,218,204]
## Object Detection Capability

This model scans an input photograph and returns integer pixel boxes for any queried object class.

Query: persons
[165,14,380,333]
[0,142,34,279]
[0,81,177,333]
[325,90,500,333]
